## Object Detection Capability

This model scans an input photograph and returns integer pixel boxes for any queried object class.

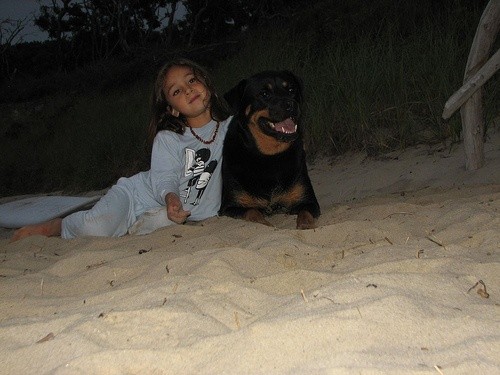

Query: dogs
[221,72,320,229]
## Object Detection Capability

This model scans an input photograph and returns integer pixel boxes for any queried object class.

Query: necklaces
[187,120,220,144]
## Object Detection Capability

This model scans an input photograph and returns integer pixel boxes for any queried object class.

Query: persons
[10,59,233,241]
